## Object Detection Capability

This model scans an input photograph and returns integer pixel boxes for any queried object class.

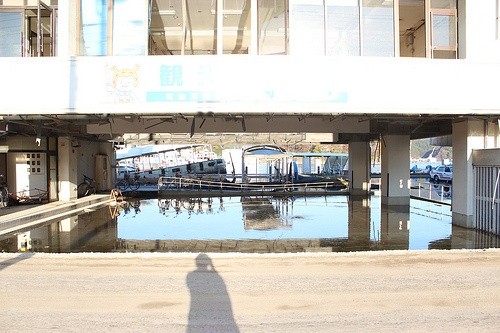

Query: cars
[429,165,453,183]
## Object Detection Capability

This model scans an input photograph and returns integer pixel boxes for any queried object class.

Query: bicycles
[130,172,140,191]
[0,174,10,208]
[77,175,96,198]
[117,171,130,190]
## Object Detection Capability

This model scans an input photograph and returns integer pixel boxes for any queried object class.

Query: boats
[408,165,431,178]
[115,144,349,182]
